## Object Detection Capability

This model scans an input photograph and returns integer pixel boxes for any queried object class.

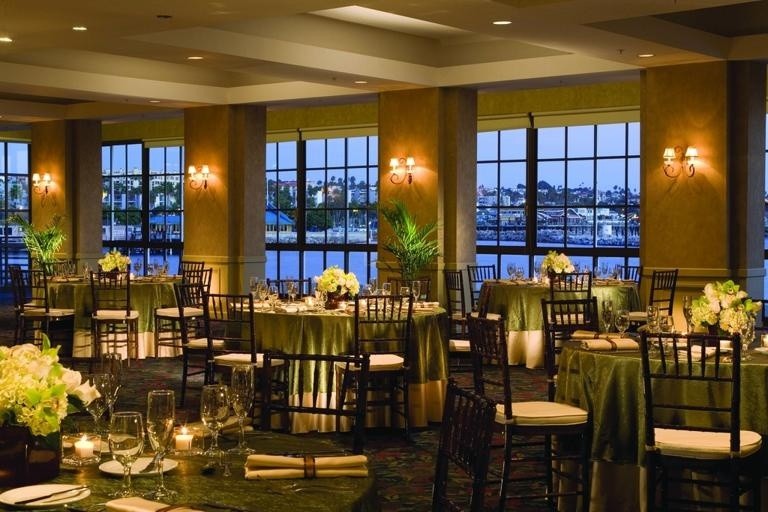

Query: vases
[324,288,344,310]
[706,325,733,347]
[0,420,37,456]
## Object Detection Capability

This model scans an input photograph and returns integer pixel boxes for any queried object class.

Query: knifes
[11,487,89,504]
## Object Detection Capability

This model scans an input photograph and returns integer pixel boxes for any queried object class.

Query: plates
[1,484,89,507]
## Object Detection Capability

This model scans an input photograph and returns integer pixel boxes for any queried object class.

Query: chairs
[336,292,412,447]
[171,281,229,408]
[385,274,432,303]
[266,276,312,300]
[639,330,767,512]
[259,350,370,456]
[465,312,592,512]
[443,251,680,402]
[4,253,214,372]
[430,378,496,512]
[203,293,289,435]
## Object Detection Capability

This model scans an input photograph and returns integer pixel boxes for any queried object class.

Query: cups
[58,431,102,467]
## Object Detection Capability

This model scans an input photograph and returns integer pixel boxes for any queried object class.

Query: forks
[257,476,356,497]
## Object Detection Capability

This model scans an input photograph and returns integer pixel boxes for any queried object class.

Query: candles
[72,435,94,457]
[175,427,194,452]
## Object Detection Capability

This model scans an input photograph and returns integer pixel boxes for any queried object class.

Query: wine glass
[53,258,169,281]
[76,352,255,502]
[601,295,768,363]
[505,261,634,283]
[248,276,432,315]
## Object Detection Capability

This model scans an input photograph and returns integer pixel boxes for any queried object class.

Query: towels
[274,304,309,313]
[676,344,717,362]
[243,454,369,481]
[404,301,440,310]
[229,300,270,308]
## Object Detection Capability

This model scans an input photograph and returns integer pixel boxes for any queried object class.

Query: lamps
[31,173,52,196]
[187,164,211,190]
[387,154,418,185]
[662,144,699,178]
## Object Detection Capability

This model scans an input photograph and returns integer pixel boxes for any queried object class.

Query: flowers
[689,278,763,335]
[0,331,102,437]
[313,264,360,298]
